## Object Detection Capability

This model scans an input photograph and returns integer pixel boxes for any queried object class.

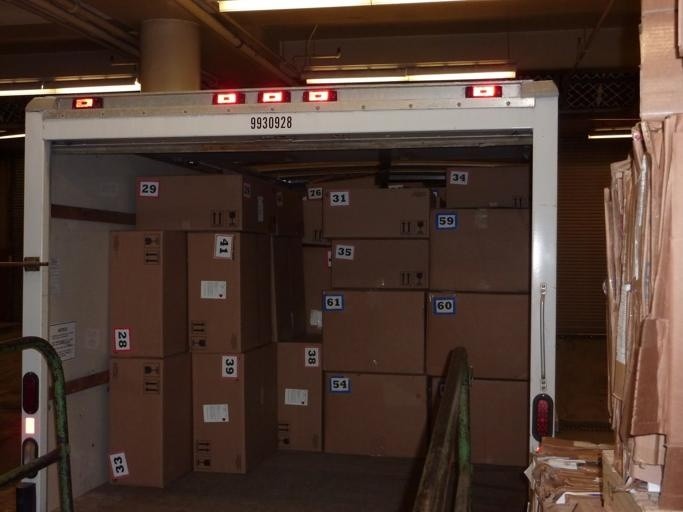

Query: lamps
[0,132,26,140]
[588,130,632,139]
[300,64,517,84]
[0,78,144,97]
